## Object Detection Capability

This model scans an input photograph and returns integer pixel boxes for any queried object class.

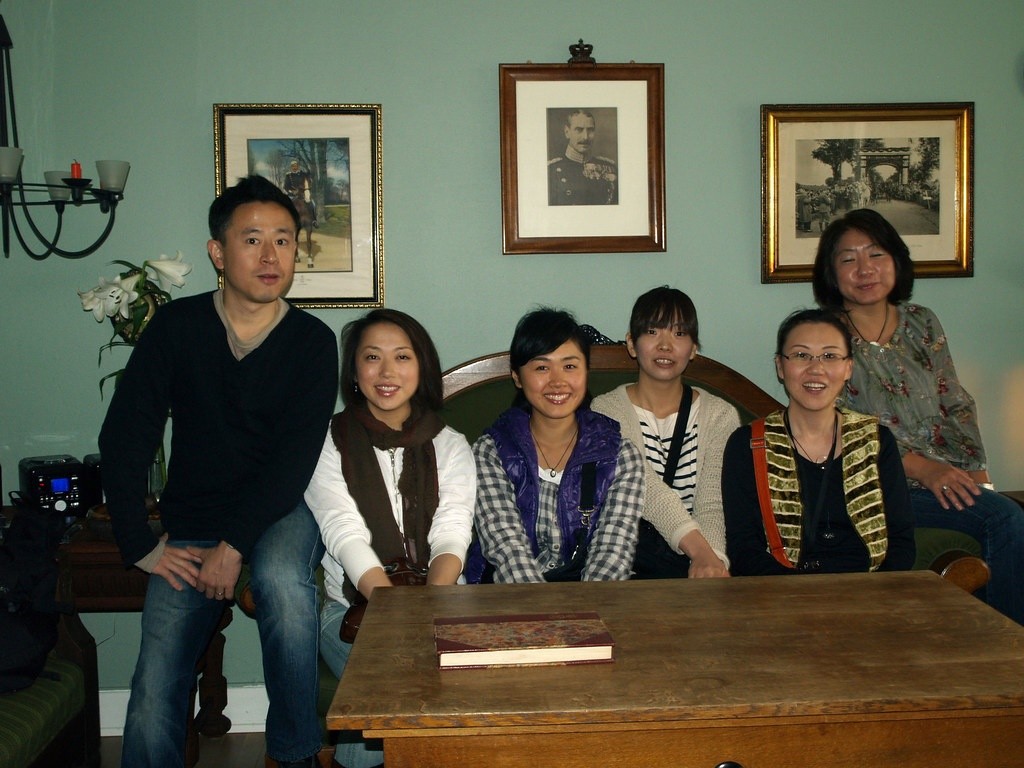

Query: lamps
[0,11,129,262]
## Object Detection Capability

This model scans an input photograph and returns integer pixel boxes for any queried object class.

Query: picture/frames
[212,100,385,312]
[760,100,975,281]
[497,58,669,256]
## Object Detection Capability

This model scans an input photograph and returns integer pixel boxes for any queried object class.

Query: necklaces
[637,390,682,449]
[842,299,888,346]
[531,426,578,477]
[785,415,837,464]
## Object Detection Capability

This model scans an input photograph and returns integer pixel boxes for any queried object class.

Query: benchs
[340,342,992,644]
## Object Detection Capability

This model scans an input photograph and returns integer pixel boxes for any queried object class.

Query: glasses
[780,352,849,368]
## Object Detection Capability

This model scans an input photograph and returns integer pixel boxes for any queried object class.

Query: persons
[593,287,740,581]
[472,306,644,590]
[721,300,915,578]
[286,161,305,199]
[810,215,1024,630]
[796,176,923,234]
[547,105,618,205]
[98,176,338,768]
[301,306,477,768]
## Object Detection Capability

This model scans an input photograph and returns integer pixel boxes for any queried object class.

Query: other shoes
[797,226,812,232]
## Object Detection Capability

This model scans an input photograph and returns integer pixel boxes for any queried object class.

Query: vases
[146,443,169,503]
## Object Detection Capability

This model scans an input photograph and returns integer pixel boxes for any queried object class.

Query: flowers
[75,247,194,404]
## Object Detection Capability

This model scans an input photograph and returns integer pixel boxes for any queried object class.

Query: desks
[325,554,1024,768]
[0,503,233,735]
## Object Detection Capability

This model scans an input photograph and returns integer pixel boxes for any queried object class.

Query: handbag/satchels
[633,517,690,580]
[0,491,61,693]
[340,561,429,644]
[543,528,597,582]
